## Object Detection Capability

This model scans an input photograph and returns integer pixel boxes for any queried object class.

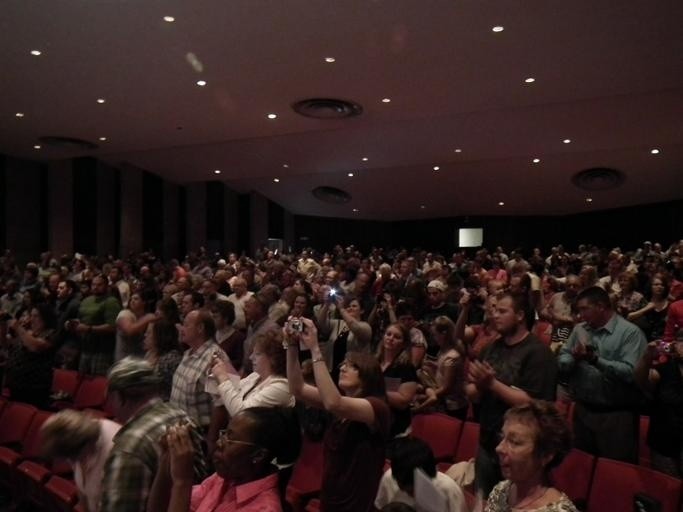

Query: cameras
[288,319,304,333]
[466,288,485,306]
[326,287,341,302]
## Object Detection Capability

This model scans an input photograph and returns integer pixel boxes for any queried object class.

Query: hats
[107,356,159,390]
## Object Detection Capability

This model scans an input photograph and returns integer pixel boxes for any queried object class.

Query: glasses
[218,429,257,451]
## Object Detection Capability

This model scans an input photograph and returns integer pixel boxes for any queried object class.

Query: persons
[1,238,683,511]
